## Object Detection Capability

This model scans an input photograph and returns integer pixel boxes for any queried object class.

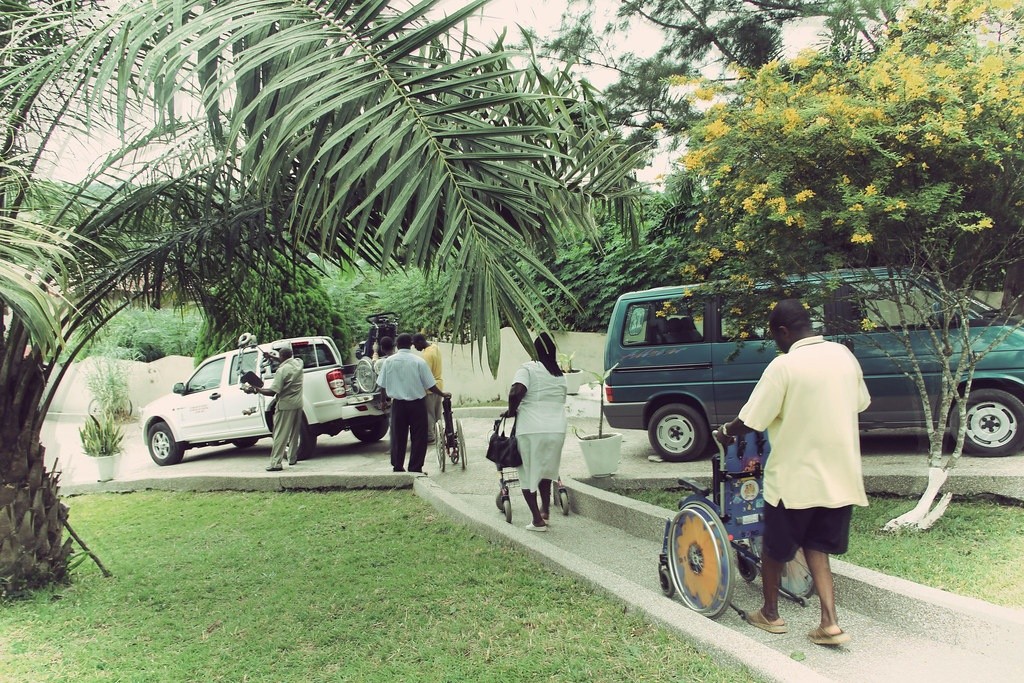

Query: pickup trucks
[136,335,392,468]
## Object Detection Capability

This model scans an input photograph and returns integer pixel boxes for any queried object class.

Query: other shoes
[384,448,392,455]
[289,455,298,465]
[265,465,283,471]
[427,439,436,444]
[393,468,406,472]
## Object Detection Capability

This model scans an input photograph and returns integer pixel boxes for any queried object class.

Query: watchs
[723,424,730,437]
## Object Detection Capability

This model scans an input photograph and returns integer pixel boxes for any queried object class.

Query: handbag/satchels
[485,410,522,468]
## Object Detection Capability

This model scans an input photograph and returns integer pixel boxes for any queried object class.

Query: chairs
[851,305,879,333]
[655,315,703,344]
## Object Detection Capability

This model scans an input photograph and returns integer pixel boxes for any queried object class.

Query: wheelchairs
[488,413,571,525]
[656,431,811,625]
[356,312,401,393]
[434,398,469,476]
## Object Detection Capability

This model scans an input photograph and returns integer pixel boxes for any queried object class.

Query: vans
[600,269,1022,460]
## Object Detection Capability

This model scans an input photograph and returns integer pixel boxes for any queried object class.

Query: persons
[716,299,871,644]
[375,336,397,375]
[241,347,304,470]
[501,332,567,531]
[377,333,451,476]
[413,333,443,445]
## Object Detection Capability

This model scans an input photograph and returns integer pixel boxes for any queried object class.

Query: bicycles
[87,397,132,419]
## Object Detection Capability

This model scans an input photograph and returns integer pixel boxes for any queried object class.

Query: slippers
[746,611,788,633]
[807,623,851,644]
[538,507,549,525]
[526,522,546,531]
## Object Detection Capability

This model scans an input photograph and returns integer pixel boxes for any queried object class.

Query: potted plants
[567,362,623,476]
[78,410,128,484]
[556,353,584,394]
